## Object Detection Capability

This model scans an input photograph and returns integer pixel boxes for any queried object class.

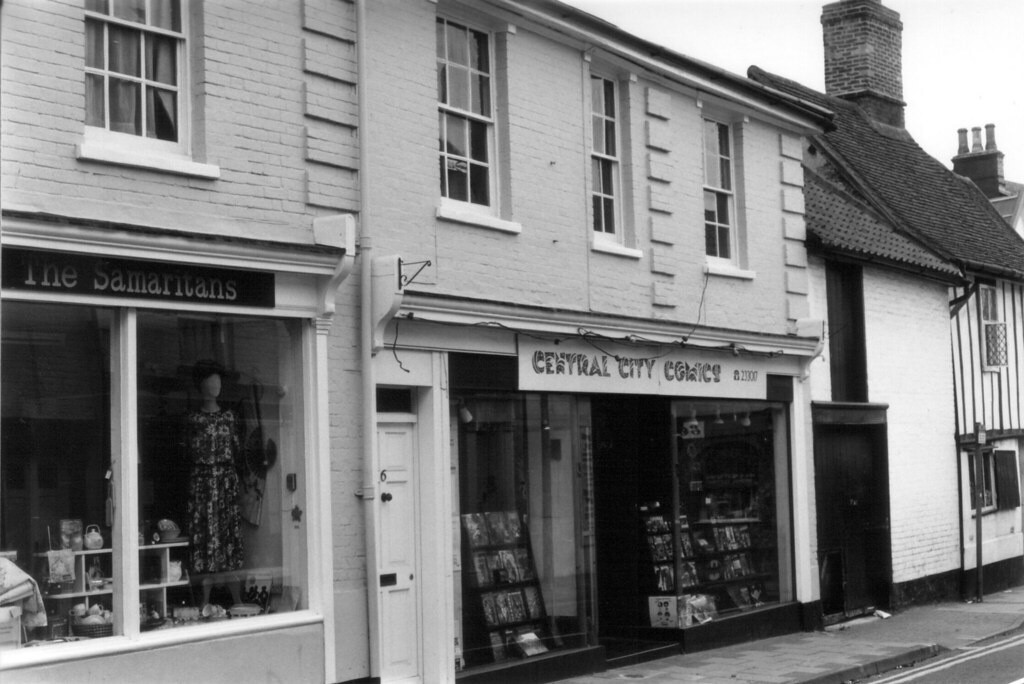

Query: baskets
[71,622,112,638]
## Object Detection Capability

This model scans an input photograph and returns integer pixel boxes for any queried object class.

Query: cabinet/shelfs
[638,514,768,626]
[35,541,189,636]
[461,511,561,669]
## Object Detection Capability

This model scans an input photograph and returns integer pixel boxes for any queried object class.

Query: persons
[186,356,245,607]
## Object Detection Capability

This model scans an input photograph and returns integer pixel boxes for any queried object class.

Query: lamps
[741,413,751,427]
[459,404,473,423]
[687,410,699,426]
[714,410,723,423]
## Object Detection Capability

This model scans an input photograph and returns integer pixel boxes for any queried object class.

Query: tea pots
[85,525,104,549]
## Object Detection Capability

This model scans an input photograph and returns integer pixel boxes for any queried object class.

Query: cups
[69,604,85,616]
[87,604,103,615]
